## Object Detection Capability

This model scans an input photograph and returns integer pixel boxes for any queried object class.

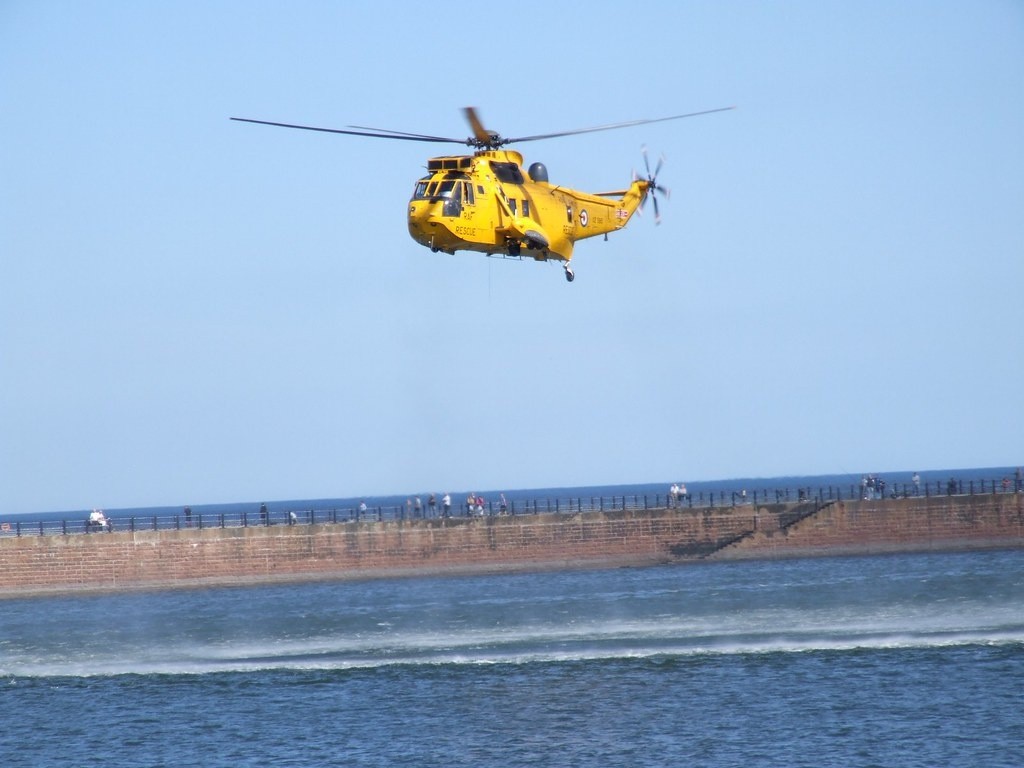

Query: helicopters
[229,100,738,287]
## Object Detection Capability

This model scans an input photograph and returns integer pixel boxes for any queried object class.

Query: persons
[184,505,192,527]
[414,496,421,517]
[497,493,507,515]
[679,483,687,507]
[429,493,437,517]
[670,483,679,508]
[950,477,958,494]
[406,497,414,518]
[912,472,920,496]
[89,508,106,532]
[359,500,367,520]
[442,493,453,518]
[859,471,882,501]
[1013,467,1023,492]
[290,512,298,524]
[260,502,268,524]
[468,492,485,517]
